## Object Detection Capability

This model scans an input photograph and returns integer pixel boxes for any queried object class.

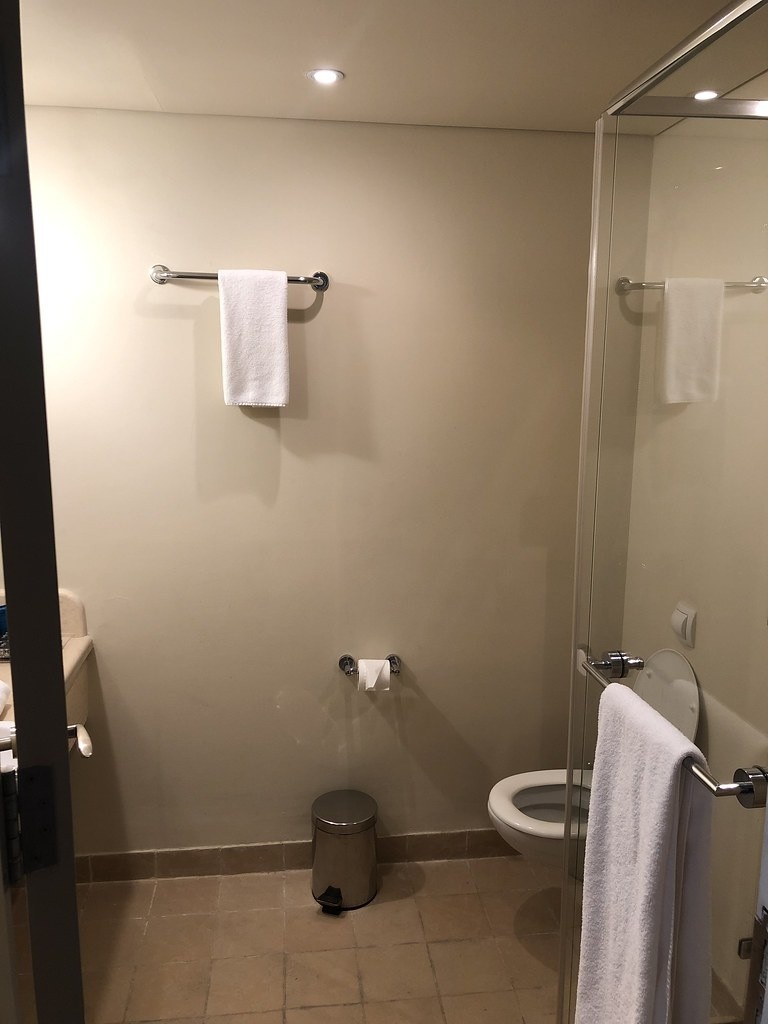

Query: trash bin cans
[311,789,378,916]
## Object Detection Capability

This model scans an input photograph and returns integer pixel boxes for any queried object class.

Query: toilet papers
[357,659,391,692]
[576,649,588,677]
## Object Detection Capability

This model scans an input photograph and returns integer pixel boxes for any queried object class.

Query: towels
[0,679,11,716]
[218,269,289,409]
[573,682,713,1024]
[655,276,725,406]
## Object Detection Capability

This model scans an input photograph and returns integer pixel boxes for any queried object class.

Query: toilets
[487,649,700,887]
